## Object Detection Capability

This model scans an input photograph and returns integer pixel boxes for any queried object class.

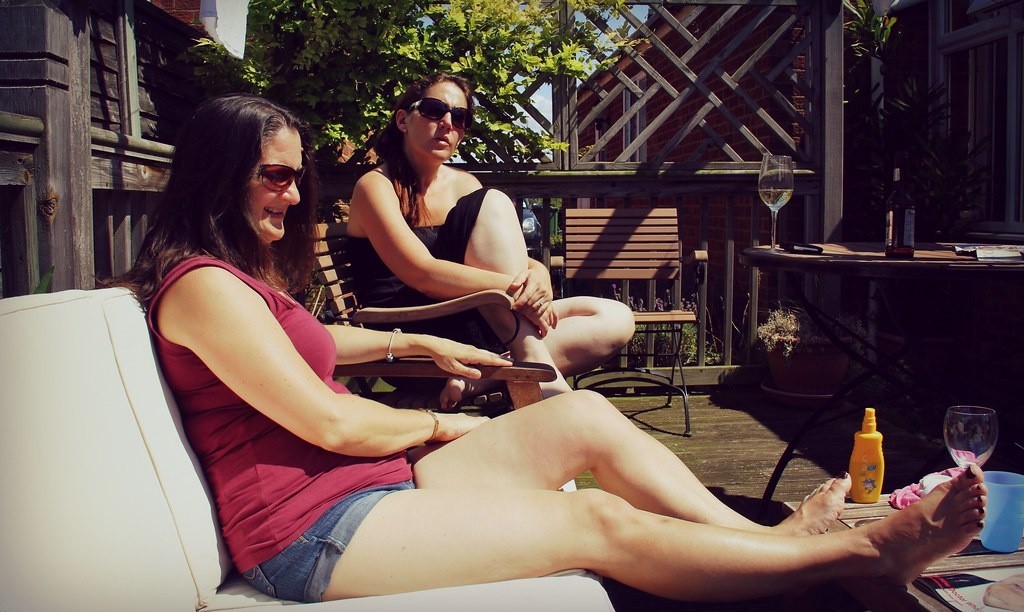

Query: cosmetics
[849,406,885,503]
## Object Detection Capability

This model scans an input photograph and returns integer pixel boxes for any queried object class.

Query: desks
[784,493,1024,612]
[742,240,1024,517]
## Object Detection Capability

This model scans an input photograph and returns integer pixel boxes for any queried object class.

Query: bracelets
[385,328,402,363]
[416,408,440,441]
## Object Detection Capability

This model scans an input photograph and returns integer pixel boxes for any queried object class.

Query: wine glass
[758,154,793,255]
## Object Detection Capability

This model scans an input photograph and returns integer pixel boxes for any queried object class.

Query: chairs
[304,220,519,411]
[565,208,708,436]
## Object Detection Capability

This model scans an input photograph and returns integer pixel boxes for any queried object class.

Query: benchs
[0,286,618,612]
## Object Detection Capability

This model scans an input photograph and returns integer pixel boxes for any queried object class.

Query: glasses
[252,163,308,193]
[407,96,474,134]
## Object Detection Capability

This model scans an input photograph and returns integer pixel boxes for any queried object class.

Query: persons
[343,69,636,413]
[916,572,1024,612]
[88,94,990,606]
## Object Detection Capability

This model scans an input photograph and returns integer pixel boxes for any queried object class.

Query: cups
[943,406,999,472]
[982,470,1024,554]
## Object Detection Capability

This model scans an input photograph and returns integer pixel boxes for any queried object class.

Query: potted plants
[759,304,870,395]
[842,0,996,384]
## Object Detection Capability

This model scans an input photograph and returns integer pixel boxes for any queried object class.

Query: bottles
[883,167,915,260]
[848,407,885,504]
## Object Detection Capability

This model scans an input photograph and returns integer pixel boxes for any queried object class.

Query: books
[912,563,1024,612]
